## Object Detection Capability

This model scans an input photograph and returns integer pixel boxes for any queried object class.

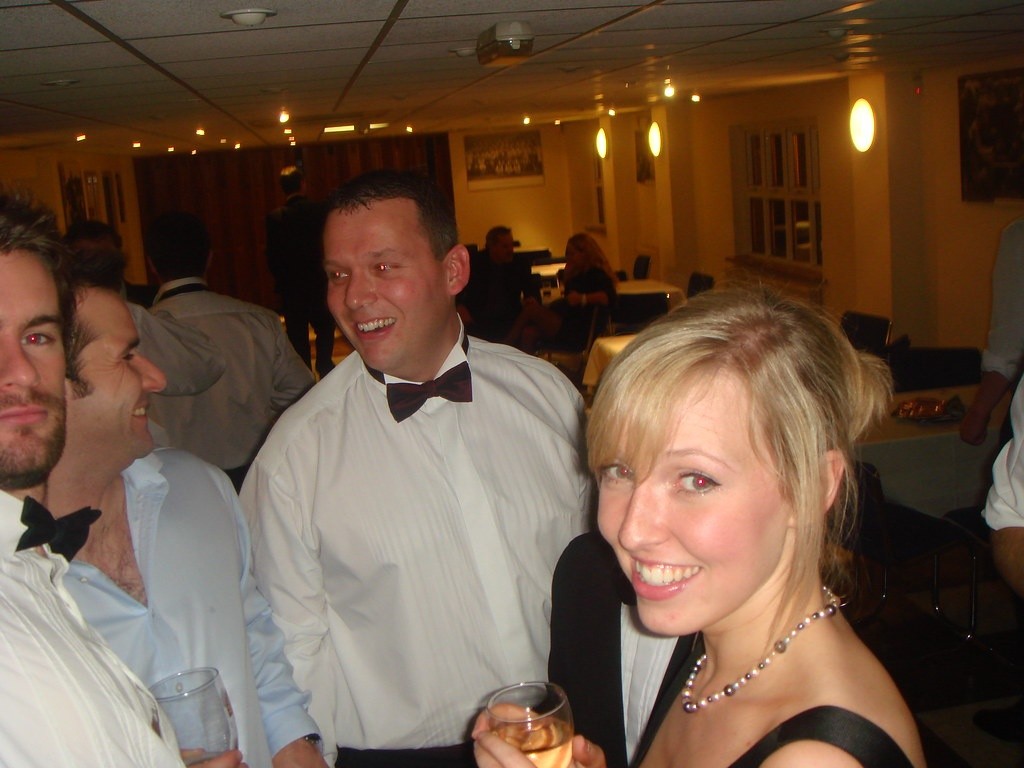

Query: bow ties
[15,494,101,564]
[363,325,473,424]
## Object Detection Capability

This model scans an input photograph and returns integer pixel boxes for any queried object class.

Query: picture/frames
[56,162,126,230]
[958,68,1024,202]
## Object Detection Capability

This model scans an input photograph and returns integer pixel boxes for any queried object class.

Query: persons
[0,184,187,768]
[464,225,543,344]
[237,165,597,768]
[981,374,1024,599]
[470,286,929,768]
[503,232,622,364]
[42,240,334,768]
[264,167,336,382]
[959,214,1024,630]
[60,210,316,495]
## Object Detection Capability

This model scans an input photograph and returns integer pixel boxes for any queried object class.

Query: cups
[147,667,237,767]
[485,682,574,768]
[543,278,551,296]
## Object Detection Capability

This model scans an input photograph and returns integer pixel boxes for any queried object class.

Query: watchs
[304,733,321,744]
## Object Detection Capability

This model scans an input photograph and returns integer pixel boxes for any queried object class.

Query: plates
[890,397,969,426]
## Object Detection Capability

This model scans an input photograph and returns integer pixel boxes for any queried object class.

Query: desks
[540,278,687,332]
[531,262,567,281]
[583,335,638,395]
[853,384,1014,520]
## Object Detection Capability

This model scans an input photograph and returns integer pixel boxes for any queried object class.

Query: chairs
[633,255,652,279]
[888,344,981,392]
[931,504,1017,672]
[537,301,611,385]
[522,250,552,265]
[609,291,671,336]
[686,270,715,297]
[837,460,980,672]
[841,311,893,354]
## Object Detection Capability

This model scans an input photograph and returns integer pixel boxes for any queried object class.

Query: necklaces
[680,585,841,713]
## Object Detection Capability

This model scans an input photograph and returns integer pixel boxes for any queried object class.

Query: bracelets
[579,293,586,304]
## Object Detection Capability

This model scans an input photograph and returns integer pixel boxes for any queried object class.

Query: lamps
[476,19,536,68]
[596,128,609,160]
[850,97,877,153]
[648,121,664,160]
